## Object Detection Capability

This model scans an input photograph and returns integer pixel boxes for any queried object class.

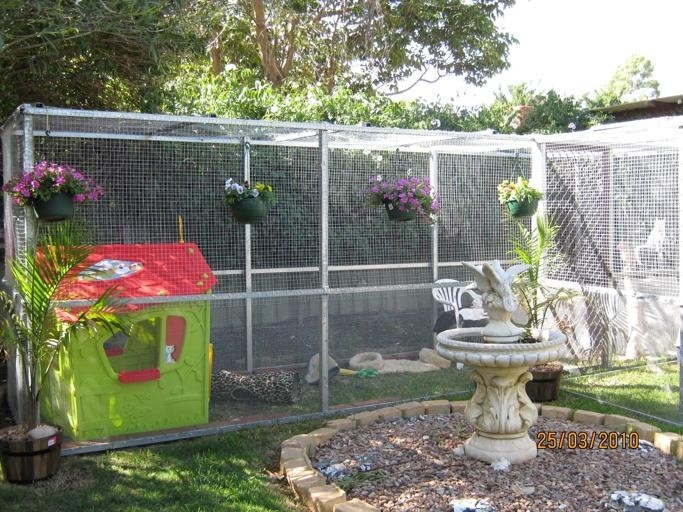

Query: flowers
[2,160,106,207]
[363,170,441,215]
[224,177,275,207]
[496,175,545,205]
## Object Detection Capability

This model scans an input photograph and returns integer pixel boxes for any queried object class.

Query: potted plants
[0,218,142,485]
[501,214,592,402]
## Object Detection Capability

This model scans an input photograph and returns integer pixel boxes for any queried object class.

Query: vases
[230,197,268,224]
[384,199,417,221]
[30,192,73,222]
[505,197,540,218]
